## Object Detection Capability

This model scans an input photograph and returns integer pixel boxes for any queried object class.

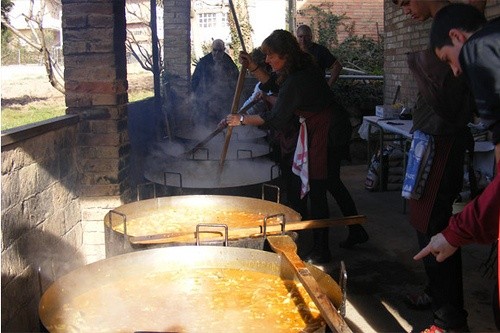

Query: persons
[391,0,485,333]
[429,2,500,137]
[191,39,240,124]
[216,47,311,221]
[226,29,369,261]
[297,25,343,88]
[413,130,500,333]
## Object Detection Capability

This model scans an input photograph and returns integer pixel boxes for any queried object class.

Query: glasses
[213,48,222,52]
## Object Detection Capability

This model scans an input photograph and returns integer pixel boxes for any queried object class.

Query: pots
[103,182,302,261]
[142,124,283,198]
[32,223,348,333]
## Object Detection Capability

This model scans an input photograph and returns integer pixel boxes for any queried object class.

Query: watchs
[239,114,244,126]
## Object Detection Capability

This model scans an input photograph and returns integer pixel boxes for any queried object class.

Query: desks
[358,115,494,212]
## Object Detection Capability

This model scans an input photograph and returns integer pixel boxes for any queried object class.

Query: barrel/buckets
[452,201,467,215]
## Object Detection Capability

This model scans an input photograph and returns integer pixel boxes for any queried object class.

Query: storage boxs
[376,105,401,119]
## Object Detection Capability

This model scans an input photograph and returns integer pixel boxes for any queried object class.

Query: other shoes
[420,324,467,333]
[302,251,331,264]
[405,291,438,310]
[339,236,369,249]
